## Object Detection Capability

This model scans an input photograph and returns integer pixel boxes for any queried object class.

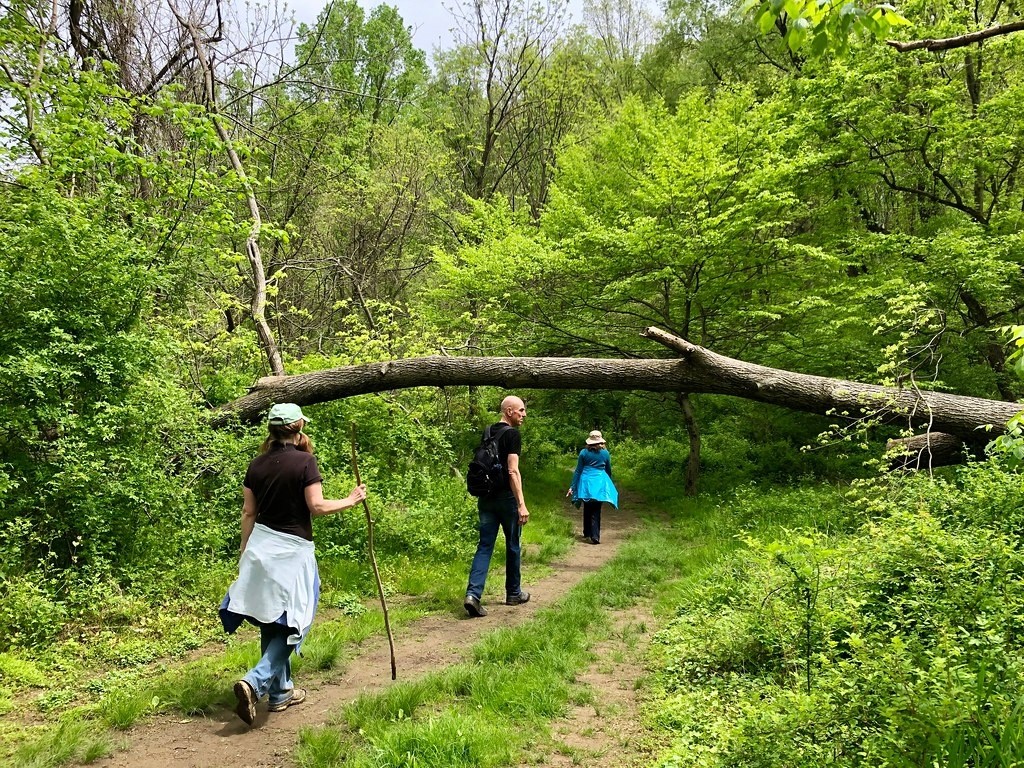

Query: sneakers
[267,690,306,711]
[234,681,258,726]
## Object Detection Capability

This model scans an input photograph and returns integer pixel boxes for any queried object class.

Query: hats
[586,430,606,444]
[268,402,310,425]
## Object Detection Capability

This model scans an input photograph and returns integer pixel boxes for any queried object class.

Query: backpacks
[467,426,514,496]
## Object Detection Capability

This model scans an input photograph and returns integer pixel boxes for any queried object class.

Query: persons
[464,396,530,617]
[566,430,612,543]
[219,403,367,724]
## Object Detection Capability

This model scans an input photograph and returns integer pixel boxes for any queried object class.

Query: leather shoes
[464,596,487,617]
[506,592,530,606]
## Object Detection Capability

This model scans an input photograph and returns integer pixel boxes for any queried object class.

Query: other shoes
[591,537,600,544]
